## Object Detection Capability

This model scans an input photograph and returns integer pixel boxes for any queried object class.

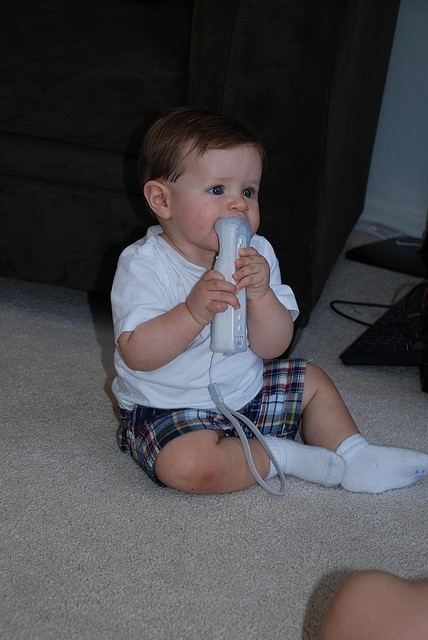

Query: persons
[110,109,428,494]
[316,569,428,640]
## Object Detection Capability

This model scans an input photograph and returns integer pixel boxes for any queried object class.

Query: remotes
[210,217,252,355]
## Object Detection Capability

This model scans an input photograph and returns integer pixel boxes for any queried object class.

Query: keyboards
[338,279,428,394]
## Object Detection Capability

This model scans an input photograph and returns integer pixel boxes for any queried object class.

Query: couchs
[1,1,401,327]
